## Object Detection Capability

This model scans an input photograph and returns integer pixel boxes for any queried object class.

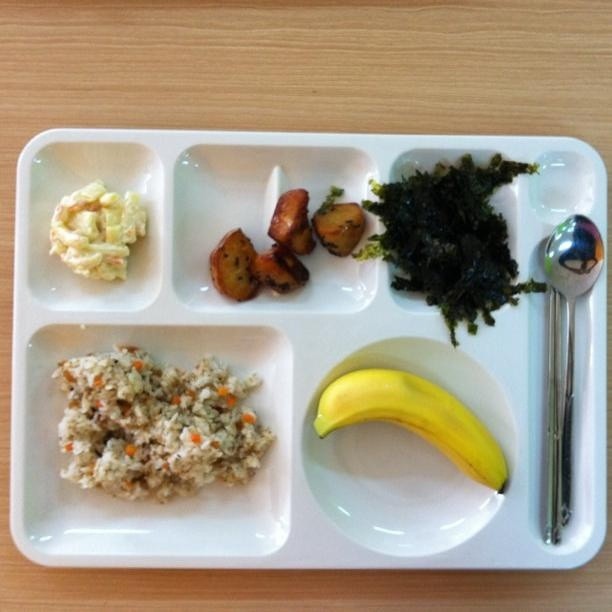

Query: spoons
[545,214,603,525]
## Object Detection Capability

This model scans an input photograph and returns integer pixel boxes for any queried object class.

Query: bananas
[313,367,509,494]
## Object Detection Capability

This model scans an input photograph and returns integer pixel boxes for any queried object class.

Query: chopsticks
[538,243,564,546]
